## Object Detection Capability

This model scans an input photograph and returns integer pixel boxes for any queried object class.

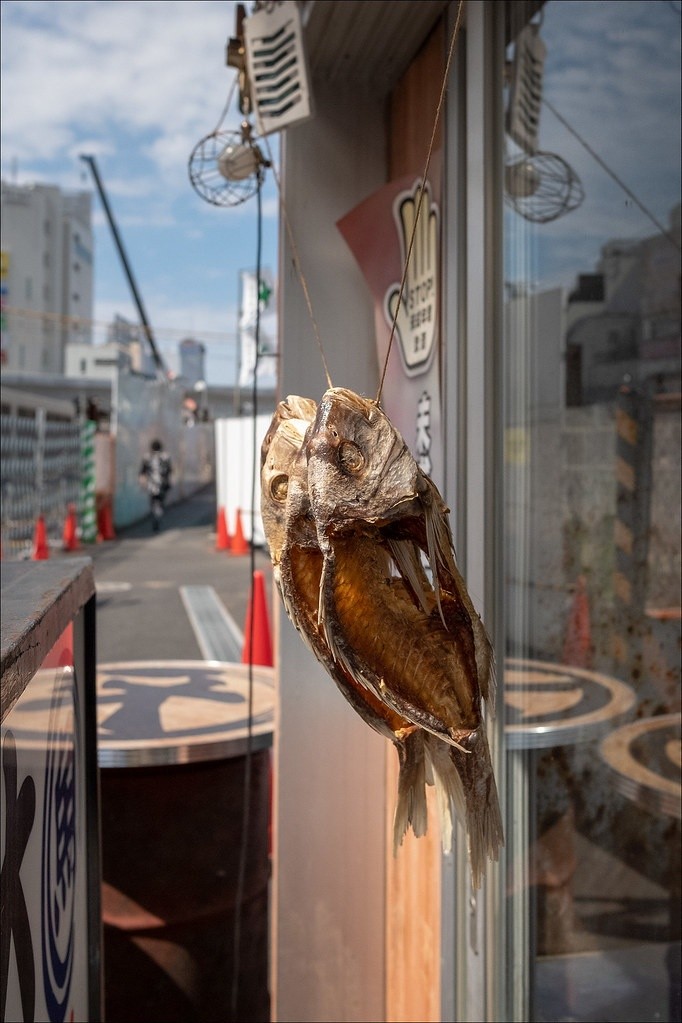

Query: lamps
[187,133,269,208]
[506,148,588,225]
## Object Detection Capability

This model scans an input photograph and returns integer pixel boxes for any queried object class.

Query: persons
[139,441,173,522]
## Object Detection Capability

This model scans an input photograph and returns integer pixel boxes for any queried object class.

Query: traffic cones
[215,506,231,549]
[61,501,79,553]
[228,508,249,557]
[34,515,51,560]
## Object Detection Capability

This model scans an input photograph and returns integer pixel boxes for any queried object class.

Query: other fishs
[258,393,433,854]
[299,385,505,892]
